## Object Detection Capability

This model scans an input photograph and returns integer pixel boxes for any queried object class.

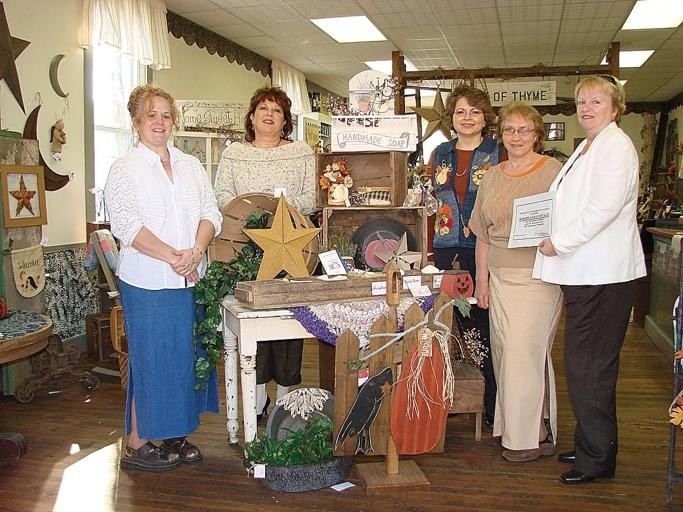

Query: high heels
[247,395,271,425]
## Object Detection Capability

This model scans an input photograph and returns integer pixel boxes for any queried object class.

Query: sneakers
[503,434,558,463]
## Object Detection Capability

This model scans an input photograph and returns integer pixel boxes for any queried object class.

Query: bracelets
[194,245,205,260]
[183,248,200,270]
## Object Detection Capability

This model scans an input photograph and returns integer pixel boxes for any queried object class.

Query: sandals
[119,441,182,472]
[158,437,202,466]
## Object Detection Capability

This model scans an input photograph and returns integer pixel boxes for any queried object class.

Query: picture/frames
[0,165,47,226]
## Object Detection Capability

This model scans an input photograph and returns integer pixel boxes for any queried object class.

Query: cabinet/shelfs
[322,208,428,272]
[314,150,405,208]
[334,297,454,456]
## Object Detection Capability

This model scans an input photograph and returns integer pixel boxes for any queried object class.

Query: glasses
[502,125,534,136]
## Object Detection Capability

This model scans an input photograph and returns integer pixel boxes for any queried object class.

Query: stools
[92,315,110,362]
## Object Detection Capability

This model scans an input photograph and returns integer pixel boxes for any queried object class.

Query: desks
[220,297,337,474]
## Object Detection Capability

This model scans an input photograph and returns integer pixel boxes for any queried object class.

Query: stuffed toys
[50,114,67,162]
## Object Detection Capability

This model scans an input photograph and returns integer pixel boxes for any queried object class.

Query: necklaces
[455,138,482,177]
[159,149,169,163]
[249,139,282,149]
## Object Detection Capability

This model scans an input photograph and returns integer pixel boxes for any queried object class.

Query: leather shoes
[560,466,618,485]
[558,451,578,463]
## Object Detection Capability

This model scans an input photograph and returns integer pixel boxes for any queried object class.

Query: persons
[532,74,647,484]
[104,85,223,473]
[468,101,564,463]
[213,84,318,424]
[419,85,509,429]
